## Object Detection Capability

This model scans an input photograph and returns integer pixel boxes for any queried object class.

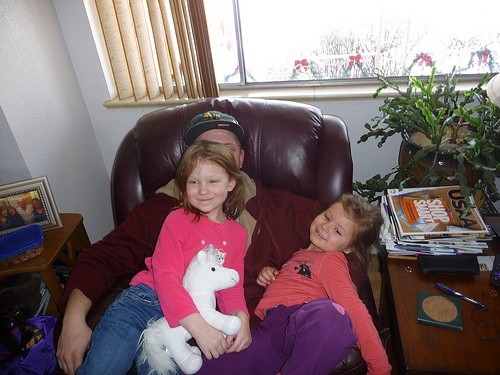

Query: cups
[488,251,500,287]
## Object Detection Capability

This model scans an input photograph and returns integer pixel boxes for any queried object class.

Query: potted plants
[345,64,500,216]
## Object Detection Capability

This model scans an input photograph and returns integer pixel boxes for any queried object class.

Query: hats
[185,110,244,149]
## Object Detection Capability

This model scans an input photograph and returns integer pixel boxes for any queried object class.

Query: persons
[16,198,35,225]
[181,191,393,375]
[0,206,26,231]
[56,110,363,375]
[31,197,47,222]
[76,139,254,375]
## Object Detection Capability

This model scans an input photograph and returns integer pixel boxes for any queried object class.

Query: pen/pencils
[436,282,484,308]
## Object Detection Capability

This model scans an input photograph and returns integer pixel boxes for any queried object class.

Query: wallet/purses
[417,252,480,276]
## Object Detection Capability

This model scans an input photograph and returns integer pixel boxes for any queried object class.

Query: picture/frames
[0,174,63,235]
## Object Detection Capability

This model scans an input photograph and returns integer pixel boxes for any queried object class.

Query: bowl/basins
[0,223,45,268]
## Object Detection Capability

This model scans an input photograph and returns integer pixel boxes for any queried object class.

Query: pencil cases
[417,254,481,275]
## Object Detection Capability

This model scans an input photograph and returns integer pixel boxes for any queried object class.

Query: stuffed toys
[135,242,240,375]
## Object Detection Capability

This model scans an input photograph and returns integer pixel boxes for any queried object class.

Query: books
[413,290,465,332]
[380,185,496,254]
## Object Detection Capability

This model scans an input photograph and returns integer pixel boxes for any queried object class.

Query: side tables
[0,213,92,318]
[376,201,500,375]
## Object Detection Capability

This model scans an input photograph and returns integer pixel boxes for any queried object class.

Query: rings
[405,267,412,272]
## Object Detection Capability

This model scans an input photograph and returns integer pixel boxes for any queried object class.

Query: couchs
[53,99,390,375]
